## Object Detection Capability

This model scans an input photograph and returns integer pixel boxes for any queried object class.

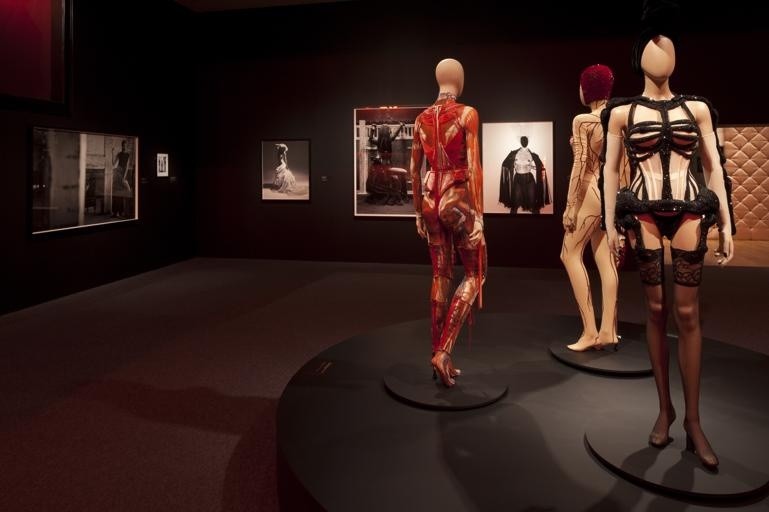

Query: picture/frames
[481,118,557,218]
[258,138,312,205]
[25,124,144,241]
[354,106,427,219]
[0,0,76,117]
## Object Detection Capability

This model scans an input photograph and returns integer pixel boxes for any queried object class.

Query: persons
[411,57,487,386]
[599,34,734,472]
[502,136,545,214]
[276,143,295,193]
[560,64,623,352]
[112,140,130,192]
